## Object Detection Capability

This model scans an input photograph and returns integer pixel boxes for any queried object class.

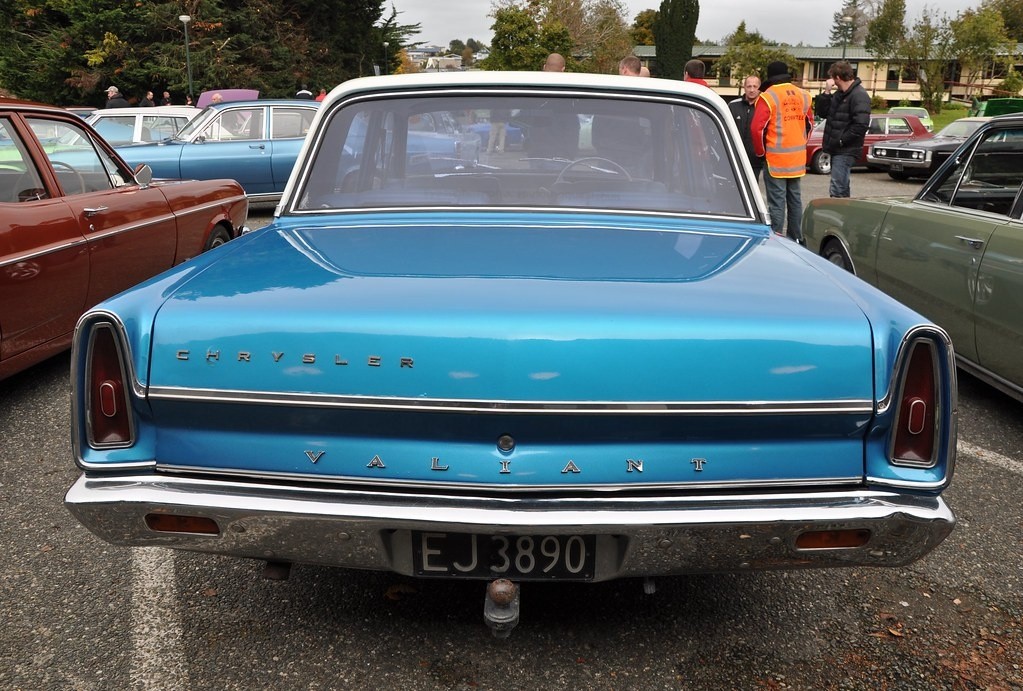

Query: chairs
[317,176,505,208]
[141,128,151,141]
[537,181,727,214]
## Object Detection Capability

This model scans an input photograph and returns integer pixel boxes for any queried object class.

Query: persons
[521,53,581,171]
[212,93,222,103]
[486,109,510,155]
[185,93,196,106]
[684,59,710,161]
[104,86,130,108]
[816,61,871,197]
[316,89,327,101]
[591,56,651,173]
[751,61,815,241]
[296,84,314,99]
[160,92,171,106]
[140,91,153,107]
[728,75,761,183]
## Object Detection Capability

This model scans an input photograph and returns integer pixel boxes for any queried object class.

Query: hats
[768,62,788,78]
[104,86,118,93]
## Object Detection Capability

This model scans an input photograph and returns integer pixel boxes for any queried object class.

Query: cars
[866,94,1023,181]
[62,71,958,642]
[884,106,934,134]
[0,89,591,209]
[800,116,1023,403]
[0,95,249,380]
[806,114,935,174]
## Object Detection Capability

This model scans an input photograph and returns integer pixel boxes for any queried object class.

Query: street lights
[178,15,193,97]
[383,41,389,75]
[843,16,852,61]
